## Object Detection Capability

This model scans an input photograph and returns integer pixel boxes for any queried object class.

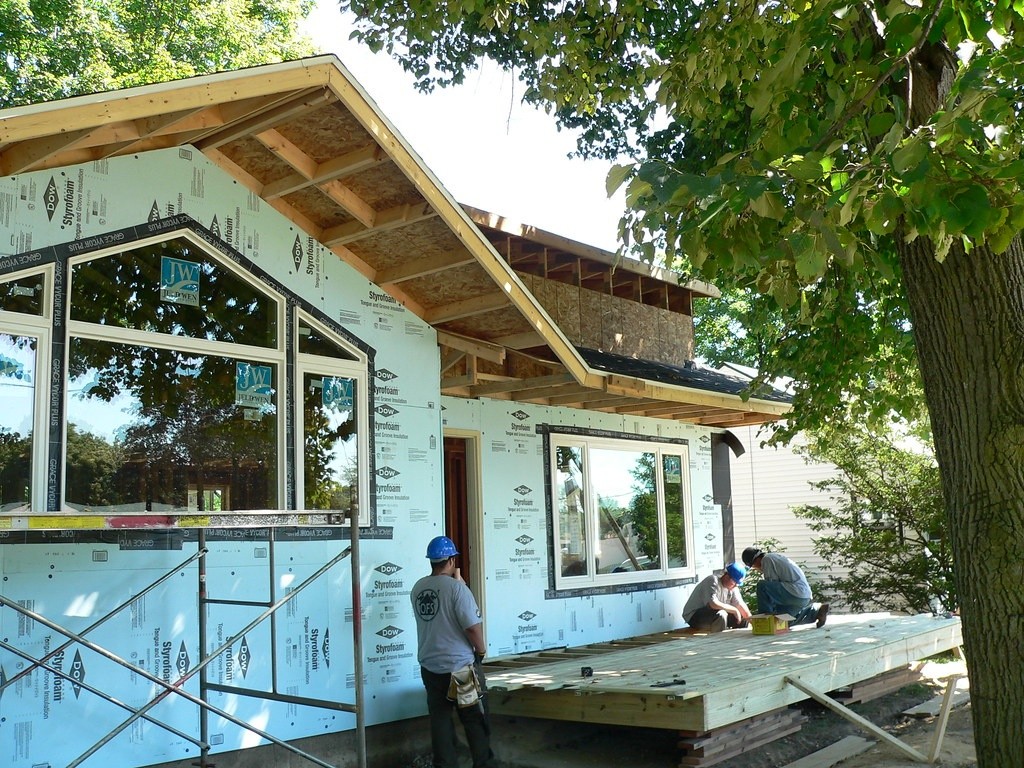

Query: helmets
[725,562,746,584]
[426,536,460,562]
[742,547,762,568]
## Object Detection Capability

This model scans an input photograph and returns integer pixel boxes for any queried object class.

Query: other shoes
[816,604,829,628]
[475,760,519,768]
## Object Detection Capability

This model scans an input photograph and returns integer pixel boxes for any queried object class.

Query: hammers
[650,679,686,687]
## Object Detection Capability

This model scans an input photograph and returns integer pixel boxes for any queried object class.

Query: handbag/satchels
[447,663,483,708]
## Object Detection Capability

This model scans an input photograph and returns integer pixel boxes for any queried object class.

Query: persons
[741,547,829,628]
[410,536,496,768]
[682,563,752,632]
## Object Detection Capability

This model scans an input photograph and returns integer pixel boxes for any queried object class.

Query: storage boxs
[748,613,796,635]
